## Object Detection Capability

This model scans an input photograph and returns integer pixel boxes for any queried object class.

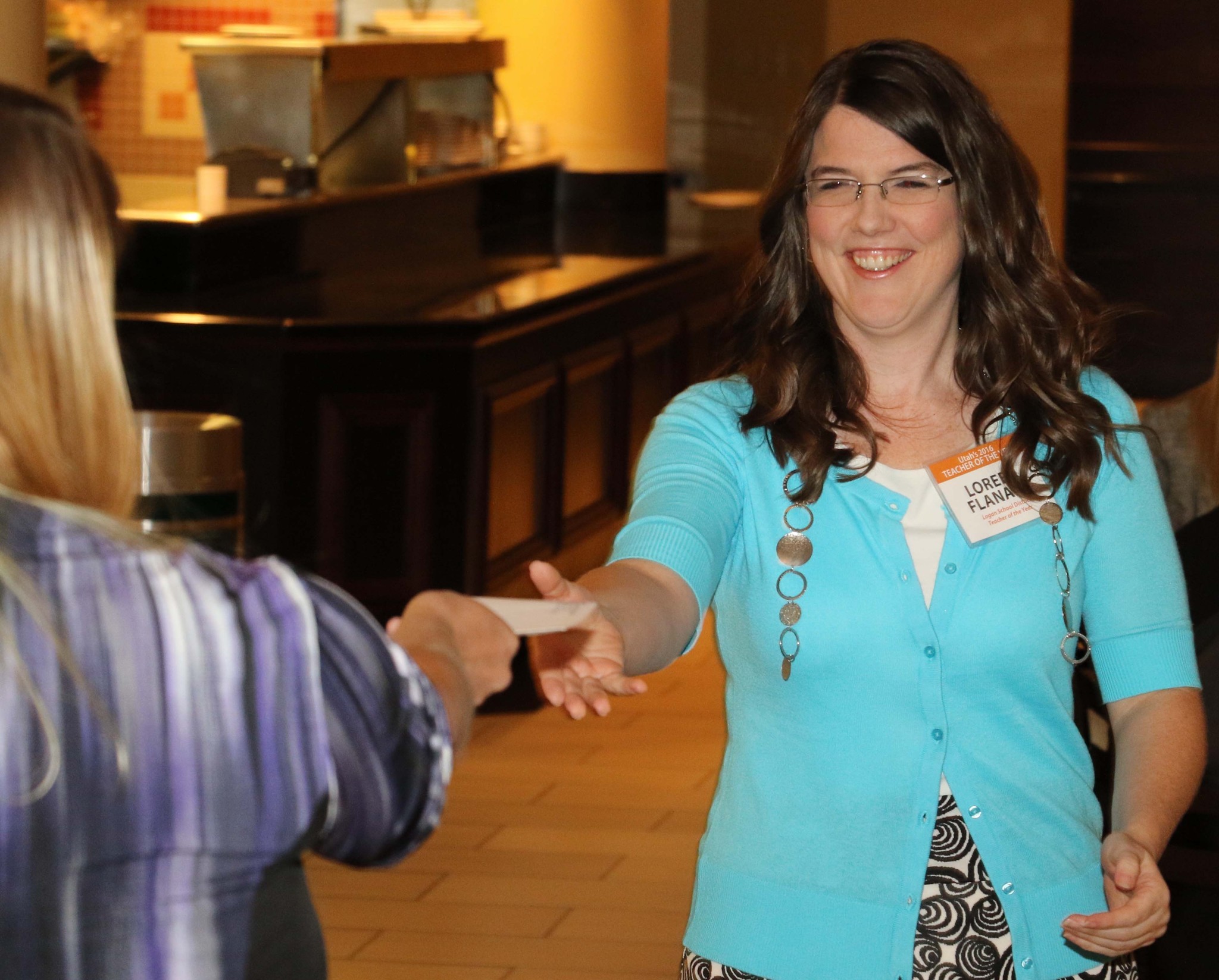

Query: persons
[531,41,1207,980]
[0,84,519,980]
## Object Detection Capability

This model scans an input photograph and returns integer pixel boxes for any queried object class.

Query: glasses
[801,177,954,208]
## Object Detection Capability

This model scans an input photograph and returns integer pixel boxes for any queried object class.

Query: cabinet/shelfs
[112,160,725,636]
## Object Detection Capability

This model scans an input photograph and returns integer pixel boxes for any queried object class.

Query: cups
[197,165,227,215]
